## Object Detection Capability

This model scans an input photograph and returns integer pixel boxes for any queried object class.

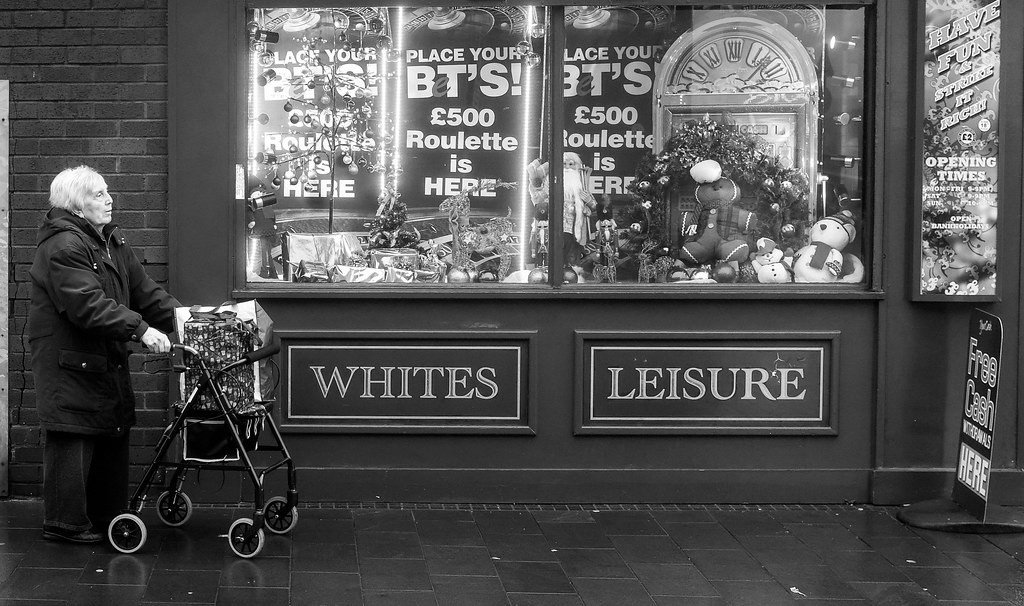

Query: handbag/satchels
[173,299,277,414]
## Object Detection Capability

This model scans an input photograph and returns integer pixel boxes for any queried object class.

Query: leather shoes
[91,519,137,535]
[42,523,103,543]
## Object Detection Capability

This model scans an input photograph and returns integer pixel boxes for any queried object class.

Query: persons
[527,152,598,266]
[28,165,183,543]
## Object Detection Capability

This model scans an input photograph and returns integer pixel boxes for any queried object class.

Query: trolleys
[105,329,300,559]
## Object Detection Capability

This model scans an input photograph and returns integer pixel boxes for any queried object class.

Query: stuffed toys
[679,160,750,268]
[749,237,793,283]
[793,209,864,283]
